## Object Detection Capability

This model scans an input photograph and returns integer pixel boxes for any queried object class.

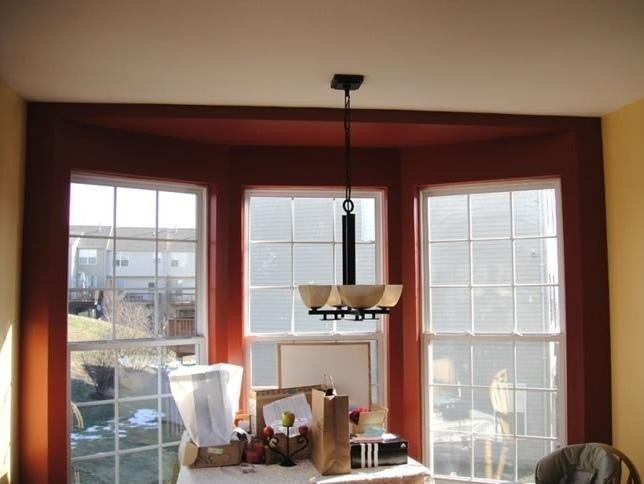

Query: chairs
[533,444,616,484]
[603,440,641,483]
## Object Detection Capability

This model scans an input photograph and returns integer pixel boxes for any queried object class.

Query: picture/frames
[278,342,371,413]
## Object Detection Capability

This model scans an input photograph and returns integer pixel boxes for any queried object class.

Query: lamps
[297,74,404,321]
